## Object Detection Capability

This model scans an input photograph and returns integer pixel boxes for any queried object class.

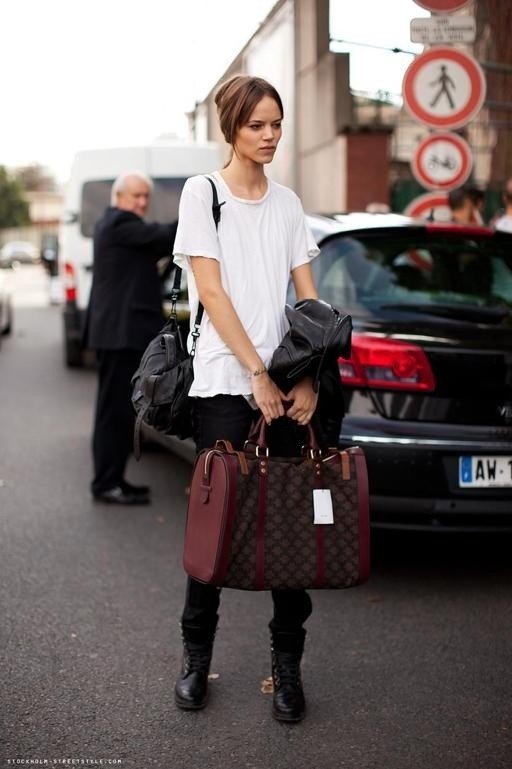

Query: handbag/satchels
[183,407,372,591]
[131,321,193,438]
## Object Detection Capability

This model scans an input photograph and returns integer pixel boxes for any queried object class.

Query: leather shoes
[95,479,152,506]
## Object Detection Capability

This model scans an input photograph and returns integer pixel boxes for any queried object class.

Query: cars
[129,211,510,562]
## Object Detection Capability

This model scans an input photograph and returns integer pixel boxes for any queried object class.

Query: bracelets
[254,367,266,376]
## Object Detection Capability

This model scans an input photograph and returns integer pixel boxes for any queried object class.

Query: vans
[53,143,248,363]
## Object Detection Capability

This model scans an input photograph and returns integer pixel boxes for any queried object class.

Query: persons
[173,76,321,721]
[448,177,512,234]
[89,168,178,504]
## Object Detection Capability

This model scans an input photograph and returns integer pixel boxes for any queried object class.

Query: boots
[270,620,306,723]
[175,622,218,710]
